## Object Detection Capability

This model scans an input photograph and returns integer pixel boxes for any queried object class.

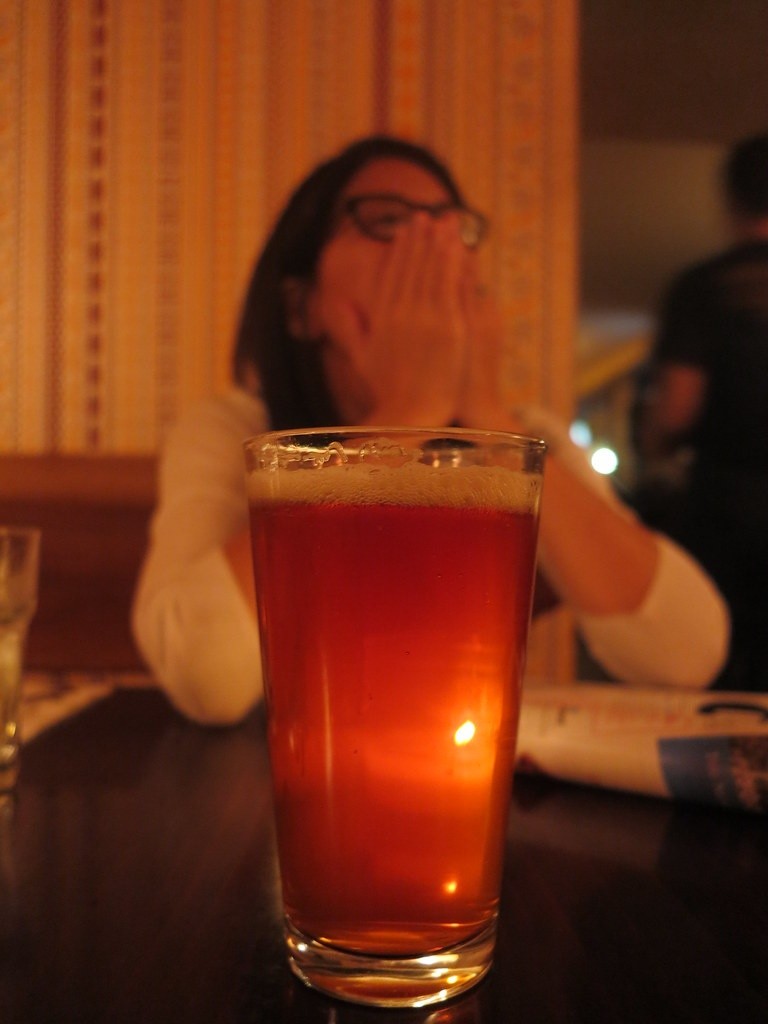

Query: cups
[239,424,548,1011]
[0,527,45,798]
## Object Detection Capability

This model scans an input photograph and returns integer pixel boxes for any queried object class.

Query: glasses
[334,194,488,249]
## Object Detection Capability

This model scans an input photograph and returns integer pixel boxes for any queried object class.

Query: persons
[638,134,768,690]
[129,133,731,725]
